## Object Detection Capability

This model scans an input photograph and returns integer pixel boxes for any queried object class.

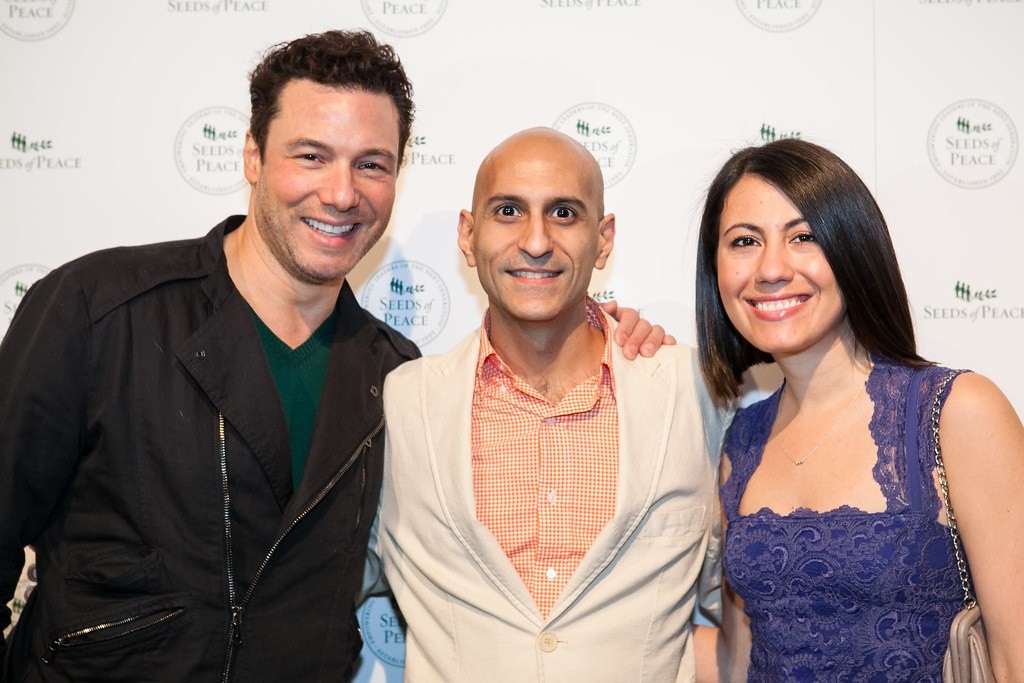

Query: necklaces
[776,363,871,466]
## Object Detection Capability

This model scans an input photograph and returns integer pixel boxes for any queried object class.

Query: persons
[352,126,733,683]
[1,28,678,683]
[694,139,1024,681]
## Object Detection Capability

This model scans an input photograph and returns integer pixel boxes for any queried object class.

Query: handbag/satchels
[931,369,1024,683]
